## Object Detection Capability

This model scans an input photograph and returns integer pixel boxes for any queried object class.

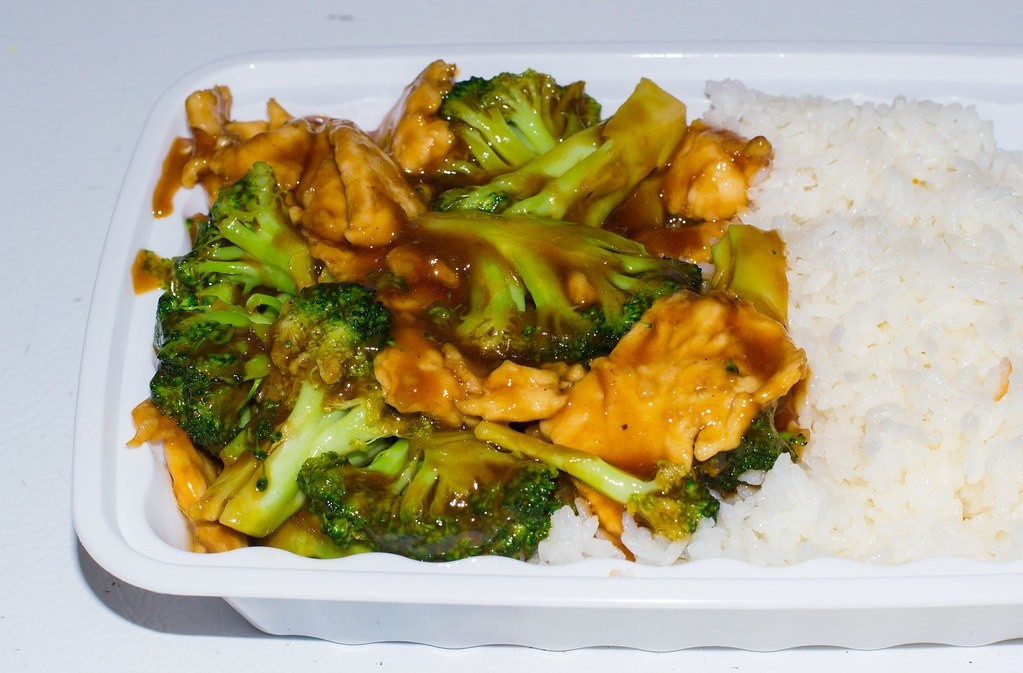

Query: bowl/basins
[70,46,1022,646]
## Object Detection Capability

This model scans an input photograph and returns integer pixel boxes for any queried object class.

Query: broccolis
[148,68,808,563]
